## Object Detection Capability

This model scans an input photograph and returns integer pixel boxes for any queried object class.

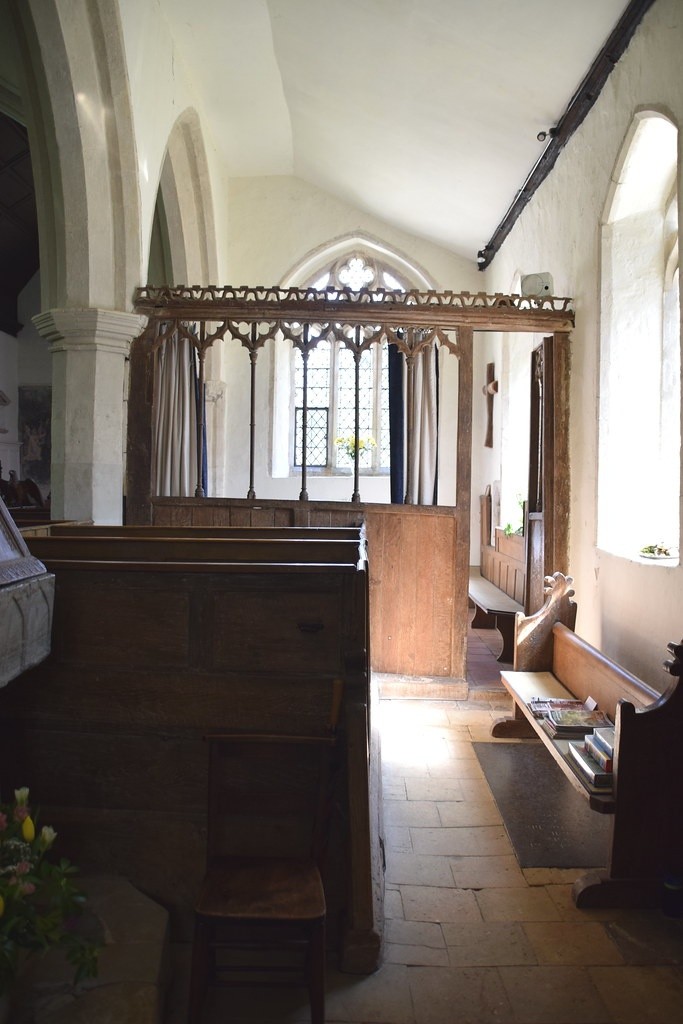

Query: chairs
[186,729,332,1024]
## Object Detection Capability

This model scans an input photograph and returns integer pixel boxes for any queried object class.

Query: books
[542,708,615,739]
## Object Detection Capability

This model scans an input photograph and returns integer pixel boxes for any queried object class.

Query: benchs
[465,527,530,661]
[492,564,683,910]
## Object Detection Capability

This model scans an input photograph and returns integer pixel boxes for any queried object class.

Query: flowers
[0,786,107,994]
